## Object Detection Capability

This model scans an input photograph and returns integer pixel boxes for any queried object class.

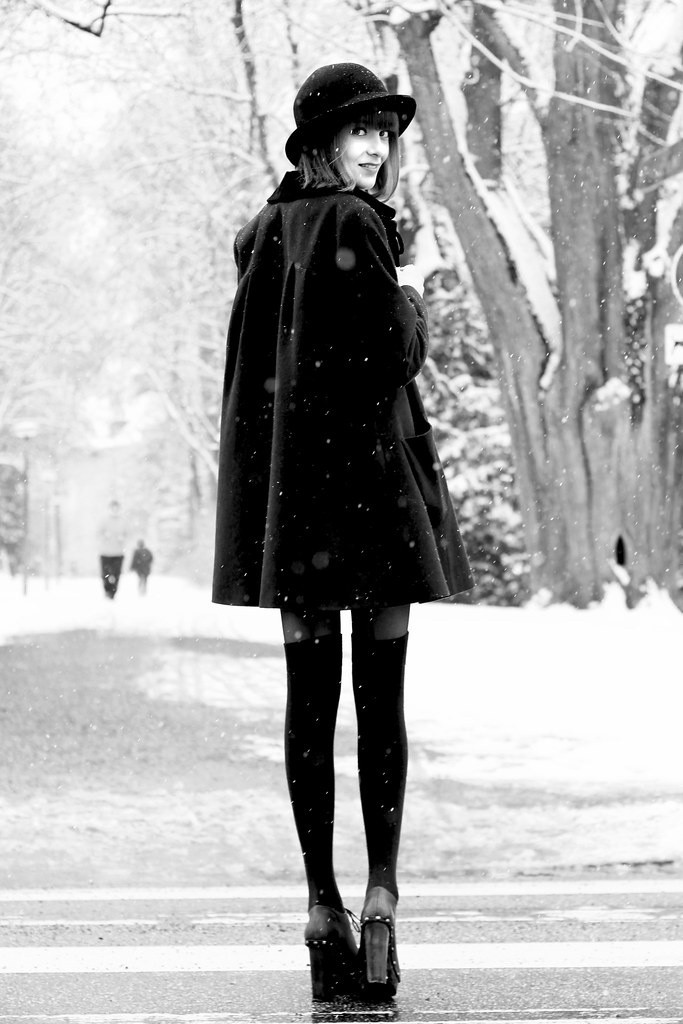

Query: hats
[285,63,417,167]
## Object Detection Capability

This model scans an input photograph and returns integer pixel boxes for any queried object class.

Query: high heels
[357,886,400,1001]
[305,905,360,1001]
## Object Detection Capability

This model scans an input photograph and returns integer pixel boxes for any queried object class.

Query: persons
[131,539,153,591]
[97,501,130,598]
[212,62,475,997]
